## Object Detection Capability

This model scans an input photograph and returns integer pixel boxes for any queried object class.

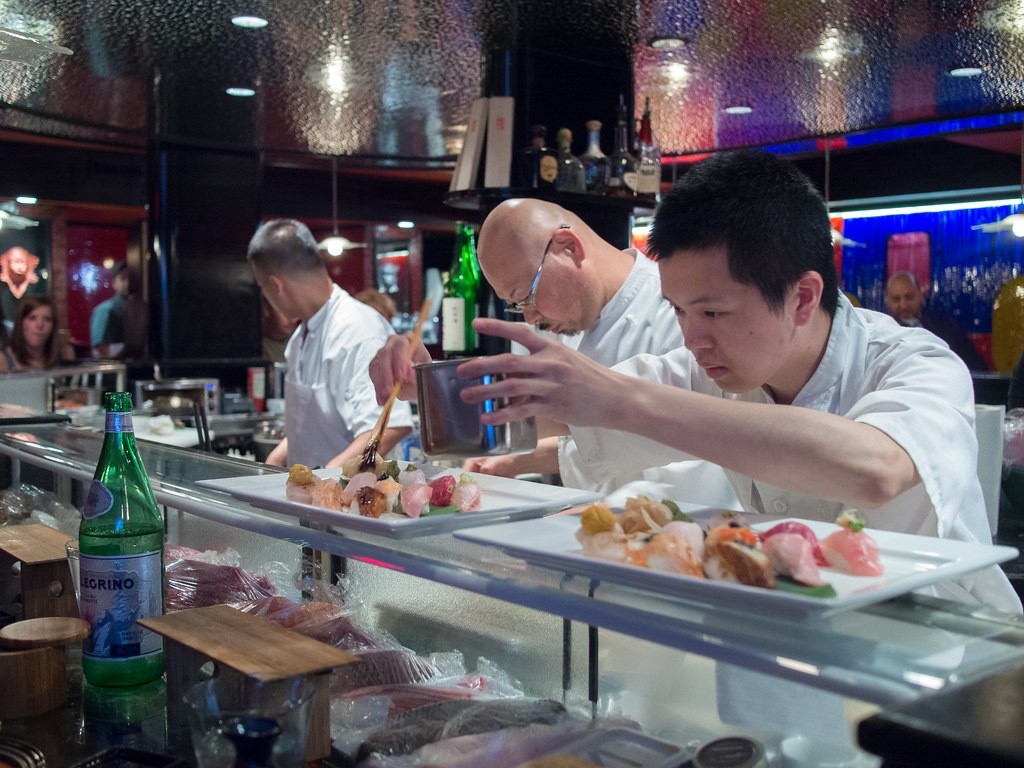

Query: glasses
[498,223,570,315]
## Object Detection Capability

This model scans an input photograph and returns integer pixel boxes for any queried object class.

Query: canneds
[246,366,265,411]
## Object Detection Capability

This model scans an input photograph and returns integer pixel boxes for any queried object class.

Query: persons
[367,146,1023,767]
[250,220,414,469]
[0,297,73,373]
[92,261,136,360]
[10,246,27,281]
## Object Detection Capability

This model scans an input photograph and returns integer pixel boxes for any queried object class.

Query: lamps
[821,135,867,249]
[318,155,368,256]
[970,211,1024,238]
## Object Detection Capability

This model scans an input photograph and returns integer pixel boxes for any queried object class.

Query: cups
[780,736,861,768]
[183,673,316,767]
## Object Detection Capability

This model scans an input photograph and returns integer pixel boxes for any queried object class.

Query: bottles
[441,220,528,358]
[77,391,167,688]
[519,97,662,197]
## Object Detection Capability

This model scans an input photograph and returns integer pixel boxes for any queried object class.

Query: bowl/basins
[412,355,538,458]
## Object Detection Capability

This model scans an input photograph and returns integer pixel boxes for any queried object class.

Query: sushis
[285,453,483,517]
[576,497,884,595]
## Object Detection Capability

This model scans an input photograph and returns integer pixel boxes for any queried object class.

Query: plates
[450,497,1022,620]
[193,459,602,540]
